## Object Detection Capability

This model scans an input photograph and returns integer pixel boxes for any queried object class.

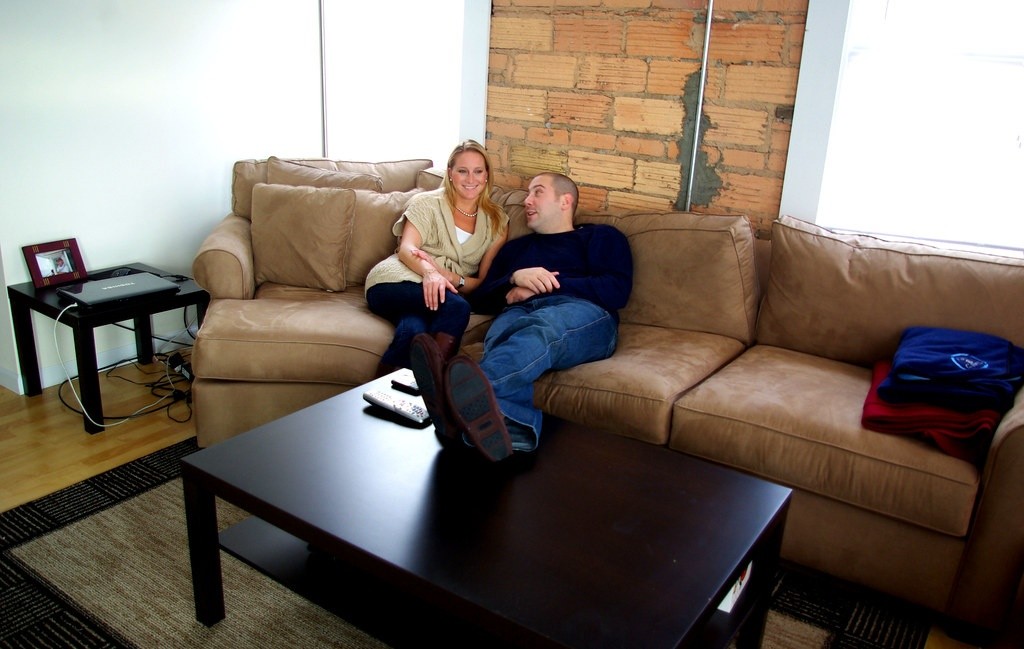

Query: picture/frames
[22,238,88,288]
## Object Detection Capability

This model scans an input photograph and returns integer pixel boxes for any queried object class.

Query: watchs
[455,276,465,289]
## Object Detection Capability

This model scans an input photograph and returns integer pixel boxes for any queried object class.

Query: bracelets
[423,269,436,275]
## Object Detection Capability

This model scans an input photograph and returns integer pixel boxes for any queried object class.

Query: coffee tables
[182,368,793,649]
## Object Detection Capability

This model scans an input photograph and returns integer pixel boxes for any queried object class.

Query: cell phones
[391,373,421,393]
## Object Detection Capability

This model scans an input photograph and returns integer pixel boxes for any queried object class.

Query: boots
[375,362,394,380]
[434,330,459,363]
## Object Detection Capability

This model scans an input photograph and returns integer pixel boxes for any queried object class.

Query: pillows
[265,156,383,193]
[344,188,426,287]
[251,183,356,290]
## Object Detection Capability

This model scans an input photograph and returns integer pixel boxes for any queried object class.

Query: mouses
[110,268,133,277]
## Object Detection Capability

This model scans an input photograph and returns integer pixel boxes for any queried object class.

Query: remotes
[363,388,430,426]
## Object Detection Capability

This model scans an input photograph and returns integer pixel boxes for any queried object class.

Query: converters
[180,364,194,382]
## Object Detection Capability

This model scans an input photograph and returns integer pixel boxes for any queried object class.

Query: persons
[360,138,510,380]
[411,172,634,463]
[55,258,70,275]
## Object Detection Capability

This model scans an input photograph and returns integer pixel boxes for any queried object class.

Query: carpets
[0,438,930,649]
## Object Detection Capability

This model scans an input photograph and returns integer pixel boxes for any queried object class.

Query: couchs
[191,186,1024,628]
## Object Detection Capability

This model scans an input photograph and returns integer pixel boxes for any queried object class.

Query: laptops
[55,271,183,310]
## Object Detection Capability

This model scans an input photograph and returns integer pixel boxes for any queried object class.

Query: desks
[7,262,210,434]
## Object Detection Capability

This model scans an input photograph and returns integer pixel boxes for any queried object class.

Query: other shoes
[412,333,461,446]
[443,355,513,464]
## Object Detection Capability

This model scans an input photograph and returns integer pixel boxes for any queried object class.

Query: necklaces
[454,205,477,217]
